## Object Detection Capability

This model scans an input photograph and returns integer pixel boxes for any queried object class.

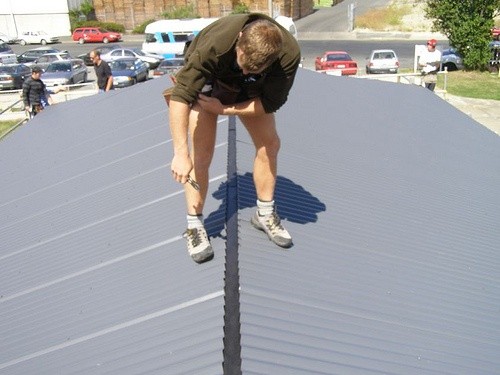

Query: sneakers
[251,206,292,247]
[182,225,213,262]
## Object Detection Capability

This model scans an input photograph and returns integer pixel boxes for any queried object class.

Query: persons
[89,50,114,94]
[170,13,301,262]
[419,39,441,92]
[23,68,49,121]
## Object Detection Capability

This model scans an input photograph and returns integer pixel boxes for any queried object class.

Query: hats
[32,67,44,73]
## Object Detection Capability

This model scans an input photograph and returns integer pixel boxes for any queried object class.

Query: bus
[140,17,220,59]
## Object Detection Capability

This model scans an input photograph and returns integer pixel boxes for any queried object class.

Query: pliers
[188,176,200,191]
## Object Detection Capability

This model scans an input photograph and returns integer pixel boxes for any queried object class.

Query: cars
[76,45,121,66]
[152,57,185,79]
[365,49,400,74]
[439,27,500,72]
[99,47,164,70]
[0,33,89,94]
[314,51,358,75]
[109,57,151,88]
[16,29,59,46]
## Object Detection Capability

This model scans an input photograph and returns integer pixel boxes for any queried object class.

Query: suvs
[72,26,123,44]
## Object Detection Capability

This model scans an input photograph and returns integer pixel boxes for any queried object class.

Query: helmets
[427,39,436,48]
